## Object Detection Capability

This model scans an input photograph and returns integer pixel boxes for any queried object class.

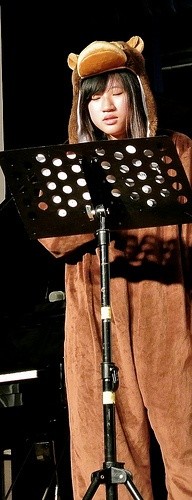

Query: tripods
[79,205,148,500]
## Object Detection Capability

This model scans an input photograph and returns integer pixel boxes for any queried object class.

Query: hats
[67,33,161,147]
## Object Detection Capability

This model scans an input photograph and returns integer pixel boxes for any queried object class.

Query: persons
[28,37,192,500]
